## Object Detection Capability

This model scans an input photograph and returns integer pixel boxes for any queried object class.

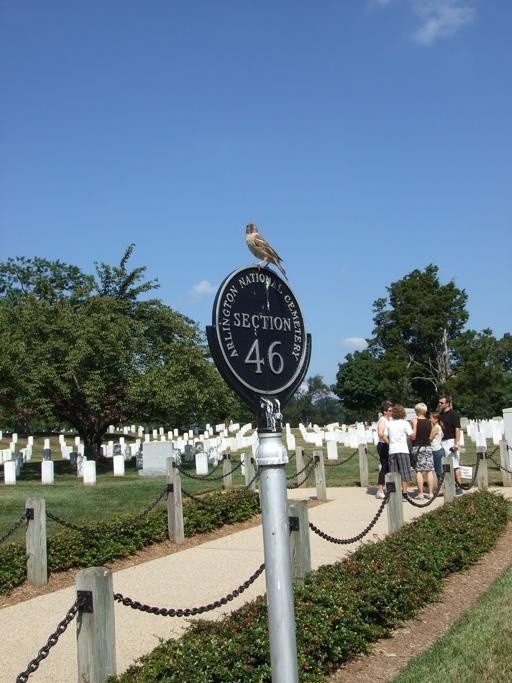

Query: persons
[434,393,462,495]
[382,403,413,496]
[427,410,446,497]
[375,401,392,499]
[410,401,434,501]
[396,419,424,492]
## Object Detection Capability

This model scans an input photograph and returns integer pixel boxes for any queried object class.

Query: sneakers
[375,490,435,501]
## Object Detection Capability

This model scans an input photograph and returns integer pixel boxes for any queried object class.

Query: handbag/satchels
[409,453,419,467]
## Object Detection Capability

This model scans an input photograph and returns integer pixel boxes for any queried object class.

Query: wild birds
[243,223,290,282]
